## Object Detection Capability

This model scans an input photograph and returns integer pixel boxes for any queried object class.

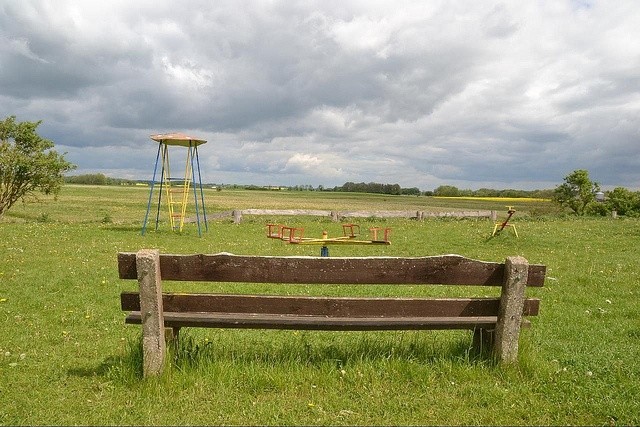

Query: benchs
[116,248,548,380]
